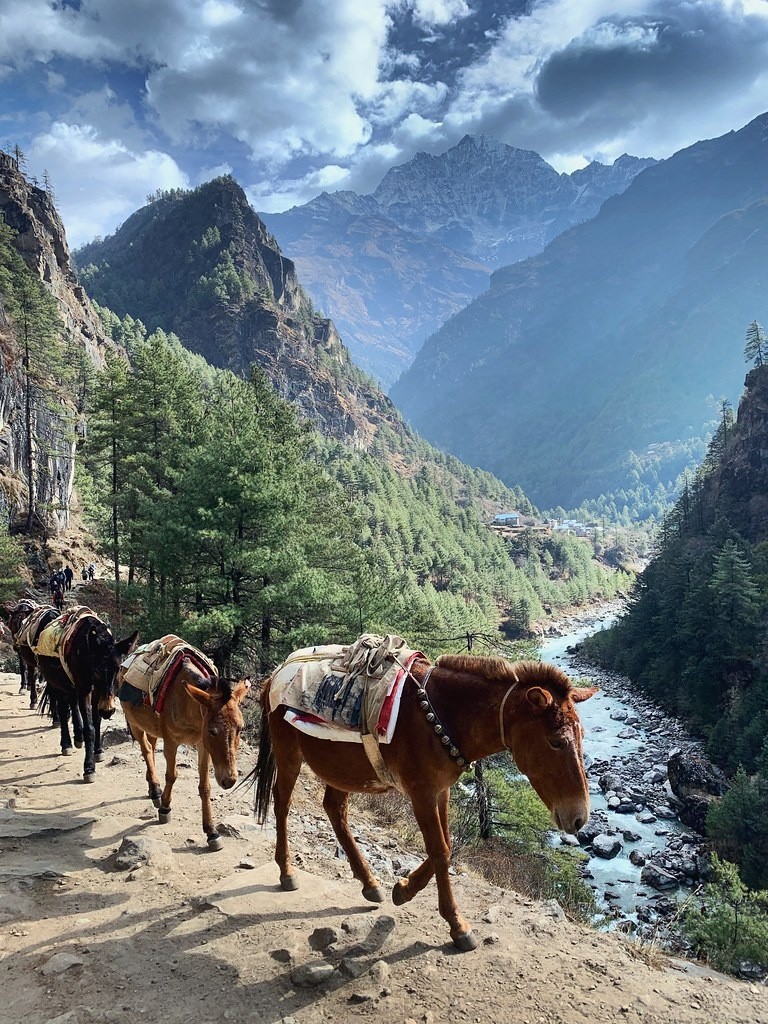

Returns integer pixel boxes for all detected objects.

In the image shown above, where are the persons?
[53,586,64,611]
[80,567,90,580]
[48,568,66,595]
[63,565,74,591]
[88,566,96,580]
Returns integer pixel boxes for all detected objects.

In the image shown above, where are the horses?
[253,633,599,951]
[0,590,138,783]
[115,633,250,851]
